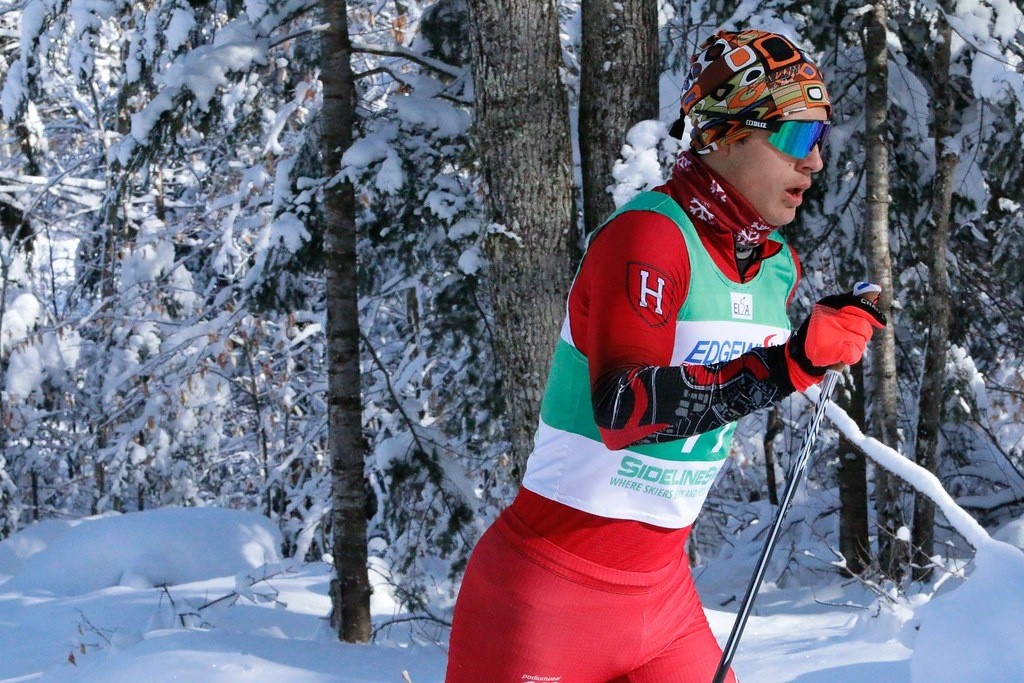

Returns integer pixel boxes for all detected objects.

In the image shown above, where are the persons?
[446,30,886,682]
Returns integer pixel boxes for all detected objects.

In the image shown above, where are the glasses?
[767,119,831,160]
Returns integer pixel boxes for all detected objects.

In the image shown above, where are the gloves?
[784,285,887,393]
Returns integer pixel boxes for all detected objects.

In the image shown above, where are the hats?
[668,30,832,154]
